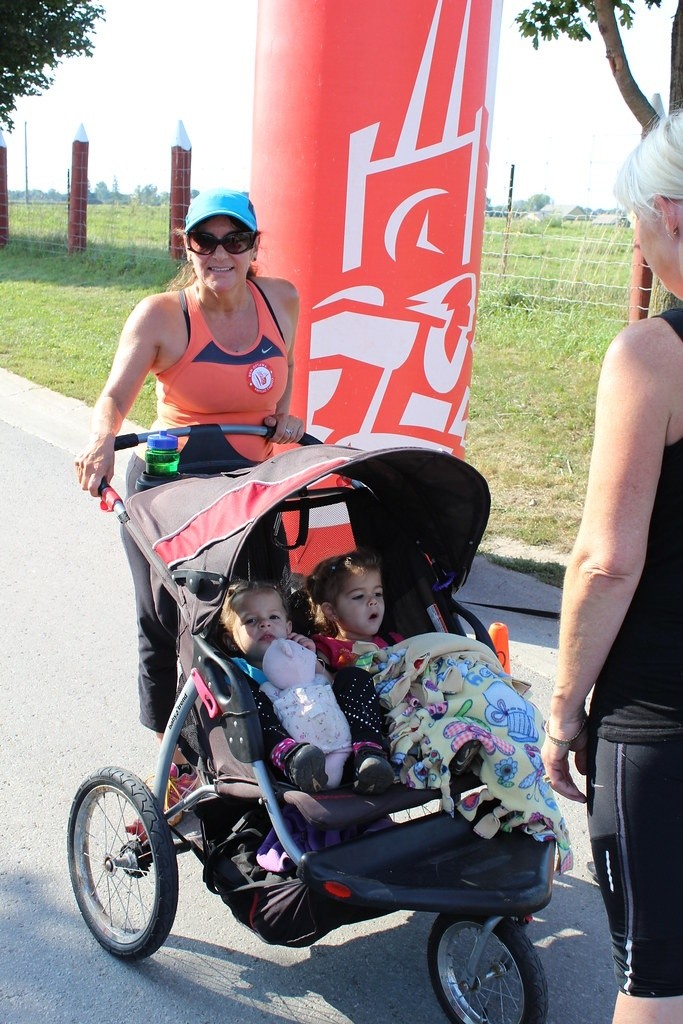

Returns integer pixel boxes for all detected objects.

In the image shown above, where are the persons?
[540,110,683,1024]
[217,550,483,796]
[75,188,304,826]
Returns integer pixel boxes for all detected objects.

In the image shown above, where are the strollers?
[66,424,555,1024]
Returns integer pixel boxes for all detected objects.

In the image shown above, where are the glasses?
[187,231,257,256]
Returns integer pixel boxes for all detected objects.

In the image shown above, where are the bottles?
[145,430,181,478]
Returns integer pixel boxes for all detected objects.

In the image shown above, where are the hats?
[185,188,257,235]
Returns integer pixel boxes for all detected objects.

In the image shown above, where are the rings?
[286,429,294,434]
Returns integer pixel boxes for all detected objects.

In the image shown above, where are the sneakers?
[167,762,200,812]
[284,742,329,793]
[450,739,482,776]
[351,746,395,796]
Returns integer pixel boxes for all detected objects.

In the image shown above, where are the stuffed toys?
[258,637,351,790]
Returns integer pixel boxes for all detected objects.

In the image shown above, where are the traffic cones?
[488,623,511,677]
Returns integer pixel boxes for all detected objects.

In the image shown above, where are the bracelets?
[318,659,325,674]
[544,713,588,747]
